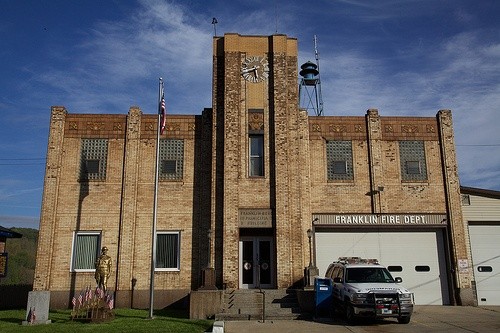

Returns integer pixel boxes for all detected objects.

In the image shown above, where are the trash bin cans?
[313,277,333,308]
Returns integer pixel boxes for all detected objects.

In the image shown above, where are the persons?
[95,246,113,295]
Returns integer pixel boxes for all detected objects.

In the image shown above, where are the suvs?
[324,257,415,322]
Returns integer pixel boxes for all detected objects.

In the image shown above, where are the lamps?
[378,185,384,214]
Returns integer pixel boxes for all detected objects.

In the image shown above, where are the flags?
[159,82,167,134]
[72,284,114,310]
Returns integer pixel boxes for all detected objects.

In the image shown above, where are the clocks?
[240,56,270,84]
